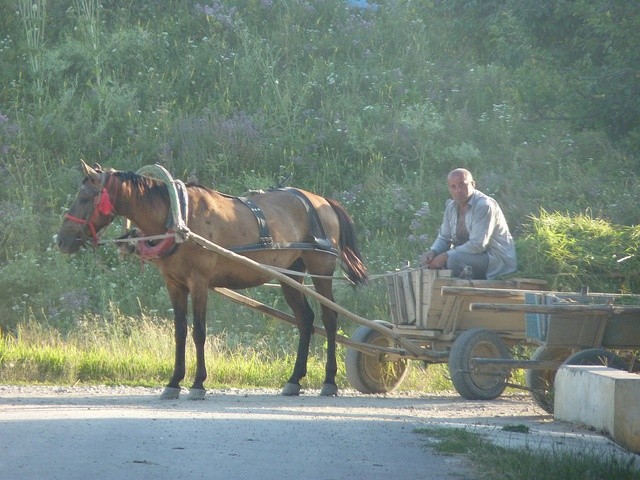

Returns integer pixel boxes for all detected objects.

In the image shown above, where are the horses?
[56,158,369,401]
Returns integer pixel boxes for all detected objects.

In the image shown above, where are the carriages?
[57,159,639,399]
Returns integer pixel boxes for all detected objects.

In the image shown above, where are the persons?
[421,168,519,280]
